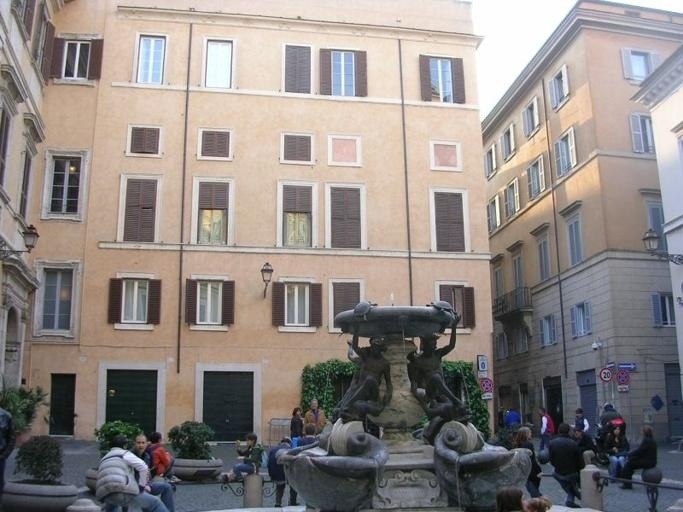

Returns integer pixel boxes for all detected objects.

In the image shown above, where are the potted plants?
[0,435,78,512]
[168,421,223,481]
[85,420,144,495]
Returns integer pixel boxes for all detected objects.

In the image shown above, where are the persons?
[267,399,327,508]
[223,433,264,482]
[0,407,16,499]
[496,402,657,512]
[406,312,466,422]
[96,430,178,512]
[330,326,393,422]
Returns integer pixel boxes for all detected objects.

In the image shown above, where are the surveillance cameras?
[592,343,598,351]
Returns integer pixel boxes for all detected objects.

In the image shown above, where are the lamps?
[642,229,683,266]
[0,225,40,260]
[261,263,273,297]
